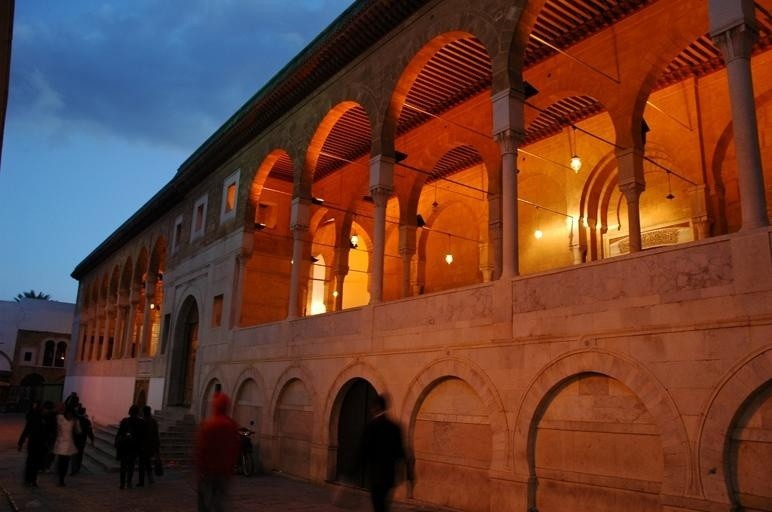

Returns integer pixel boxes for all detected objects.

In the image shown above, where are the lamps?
[445,235,453,264]
[351,214,358,247]
[431,182,439,213]
[667,171,675,200]
[570,126,582,174]
[534,206,543,240]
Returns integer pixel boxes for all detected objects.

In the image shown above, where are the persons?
[191,392,241,512]
[363,395,418,512]
[17,393,95,487]
[137,406,161,487]
[113,405,142,490]
